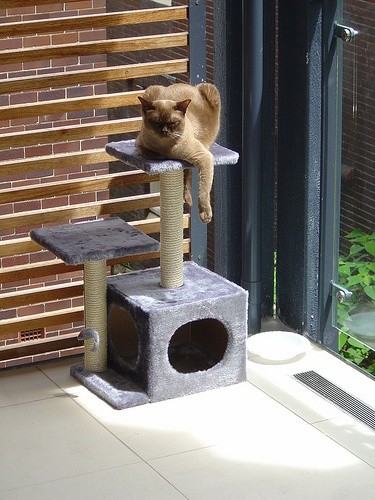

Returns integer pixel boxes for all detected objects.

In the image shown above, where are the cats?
[135,82,221,224]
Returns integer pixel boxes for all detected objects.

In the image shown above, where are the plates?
[246,331,311,360]
